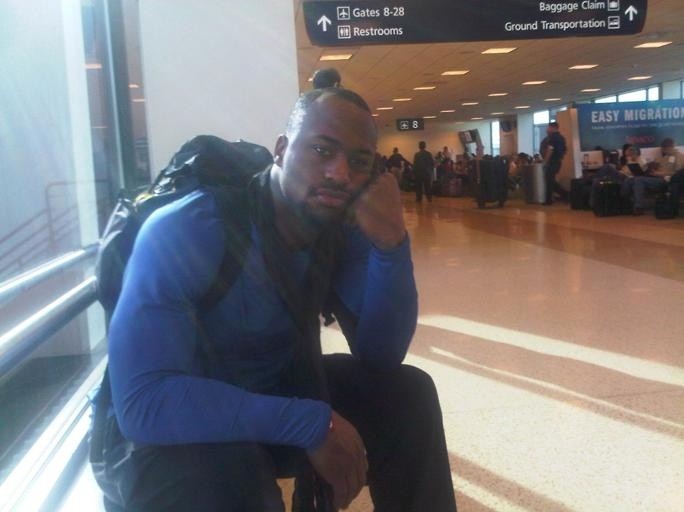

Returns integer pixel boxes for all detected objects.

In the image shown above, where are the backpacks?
[91,133,347,462]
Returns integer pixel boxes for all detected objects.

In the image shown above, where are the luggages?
[570,153,593,210]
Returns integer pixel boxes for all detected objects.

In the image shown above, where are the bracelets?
[328,404,337,430]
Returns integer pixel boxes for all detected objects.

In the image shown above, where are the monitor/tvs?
[500,121,512,133]
[462,132,476,143]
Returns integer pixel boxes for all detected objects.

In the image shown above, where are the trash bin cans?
[522,163,546,205]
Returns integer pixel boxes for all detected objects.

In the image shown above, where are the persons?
[345,28,348,36]
[616,138,682,215]
[87,88,461,512]
[375,141,541,203]
[540,121,567,204]
[339,28,343,36]
[312,67,340,89]
